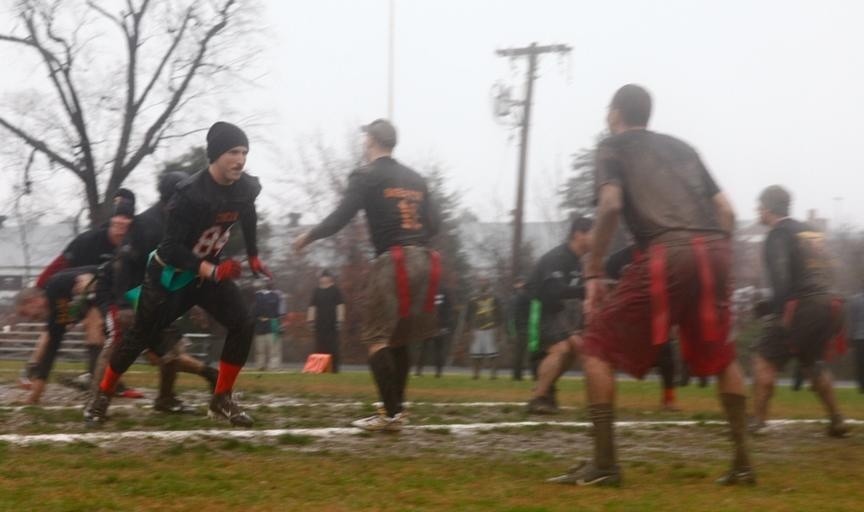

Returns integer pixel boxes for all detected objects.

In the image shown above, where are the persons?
[654,337,684,414]
[305,268,347,373]
[513,217,597,413]
[463,282,505,380]
[288,118,441,431]
[745,184,851,436]
[503,276,543,381]
[412,282,454,377]
[542,82,755,488]
[844,279,864,396]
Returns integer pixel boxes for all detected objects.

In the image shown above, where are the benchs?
[0,322,214,364]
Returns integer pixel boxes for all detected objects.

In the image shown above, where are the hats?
[111,188,134,202]
[205,121,249,166]
[157,172,188,198]
[359,119,398,149]
[109,205,133,220]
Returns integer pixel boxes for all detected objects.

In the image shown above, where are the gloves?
[248,256,272,280]
[211,260,245,283]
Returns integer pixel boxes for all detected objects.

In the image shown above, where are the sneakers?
[659,403,859,438]
[150,397,183,413]
[14,368,146,400]
[351,408,406,432]
[546,464,621,488]
[82,389,111,423]
[712,470,757,488]
[205,395,254,427]
[524,386,564,415]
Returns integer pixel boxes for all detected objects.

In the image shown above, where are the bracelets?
[582,274,604,281]
